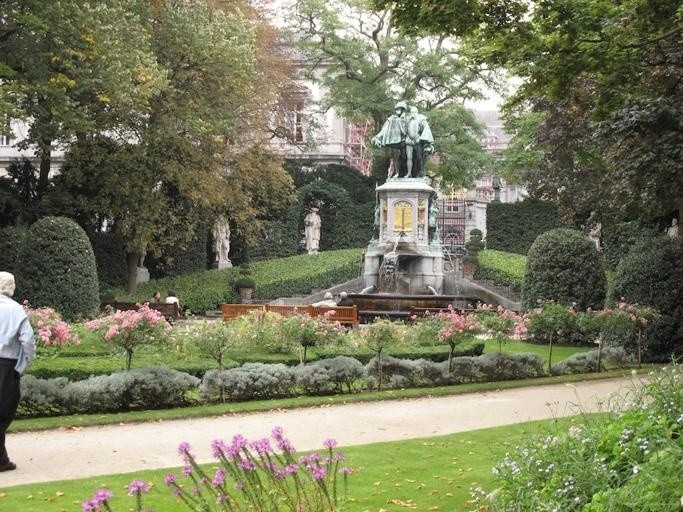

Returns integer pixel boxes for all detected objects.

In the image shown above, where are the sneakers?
[0,462,16,471]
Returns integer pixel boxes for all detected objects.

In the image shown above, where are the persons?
[213,212,232,263]
[406,106,433,178]
[590,211,602,251]
[0,271,36,470]
[371,101,407,177]
[338,292,360,325]
[304,206,322,255]
[150,292,160,303]
[309,292,336,307]
[668,217,679,238]
[166,289,180,312]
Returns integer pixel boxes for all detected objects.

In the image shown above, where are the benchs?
[100,301,188,325]
[457,310,519,317]
[311,304,362,328]
[406,306,457,324]
[220,301,266,323]
[358,311,410,325]
[266,302,313,320]
